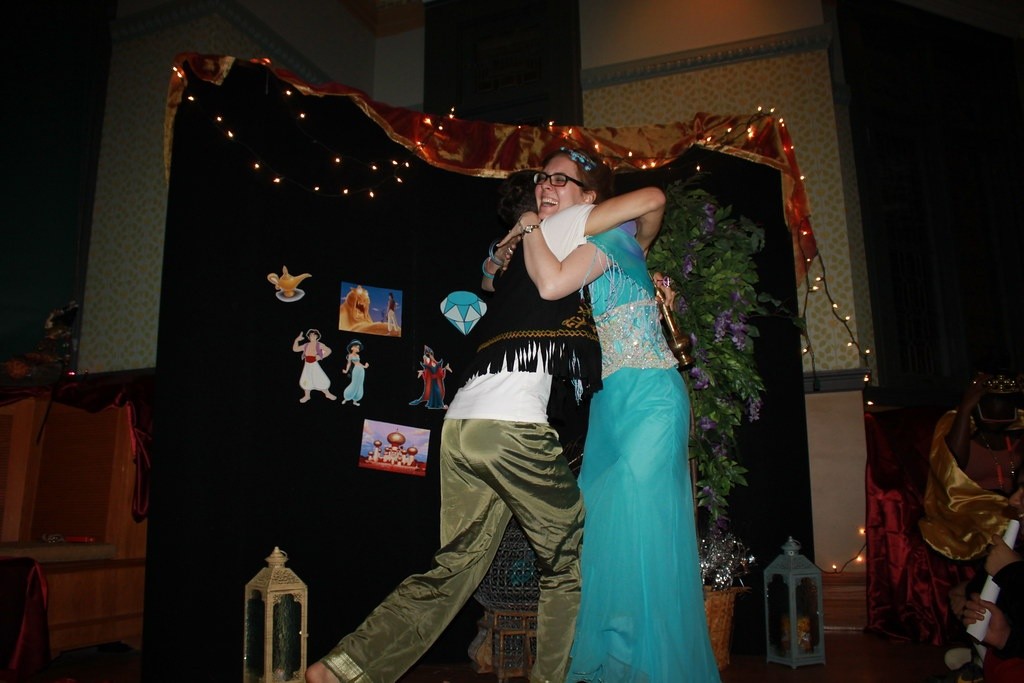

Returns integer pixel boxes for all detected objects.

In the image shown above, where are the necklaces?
[959,462,1024,682]
[972,423,1016,494]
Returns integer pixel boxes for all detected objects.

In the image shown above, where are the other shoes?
[943,642,987,672]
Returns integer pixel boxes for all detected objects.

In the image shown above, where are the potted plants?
[641,175,799,680]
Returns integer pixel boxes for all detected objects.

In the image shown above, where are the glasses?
[534,172,584,188]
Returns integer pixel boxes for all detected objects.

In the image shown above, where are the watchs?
[521,223,541,239]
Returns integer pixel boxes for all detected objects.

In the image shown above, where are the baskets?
[701,585,752,673]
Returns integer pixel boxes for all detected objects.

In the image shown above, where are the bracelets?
[488,238,505,266]
[480,256,496,279]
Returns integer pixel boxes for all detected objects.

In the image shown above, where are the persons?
[918,371,1024,567]
[303,165,670,682]
[493,147,722,683]
